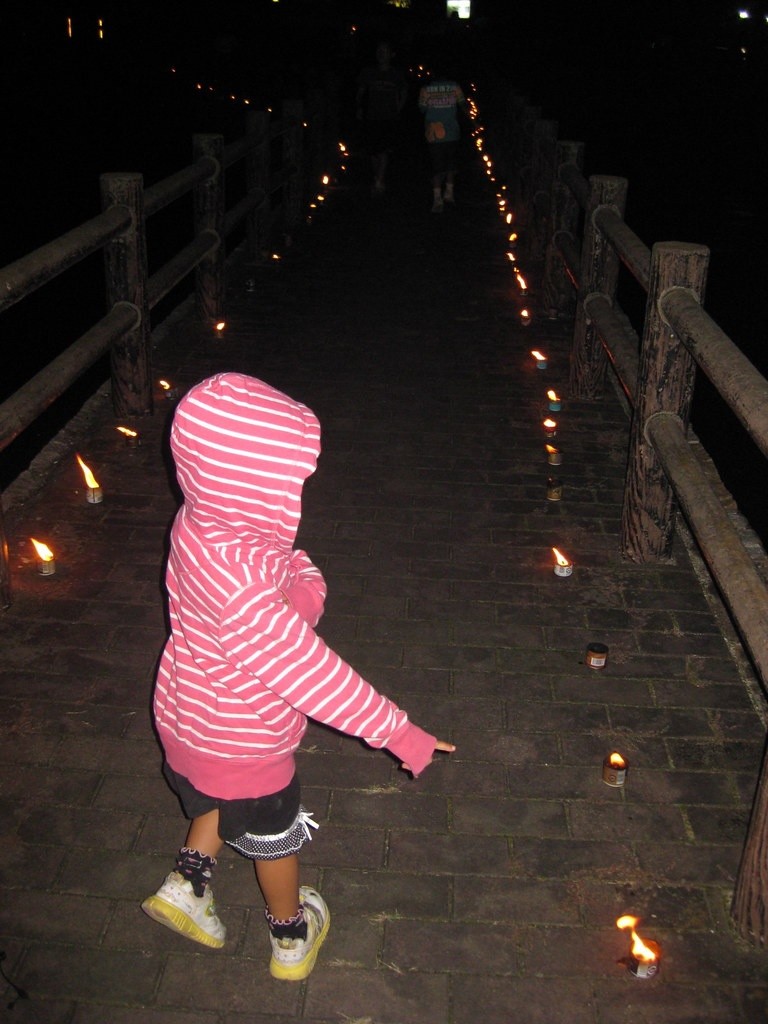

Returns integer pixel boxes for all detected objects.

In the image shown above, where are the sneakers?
[141,872,229,949]
[270,885,332,980]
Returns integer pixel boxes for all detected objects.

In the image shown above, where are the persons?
[418,63,467,215]
[355,37,413,196]
[141,371,456,982]
